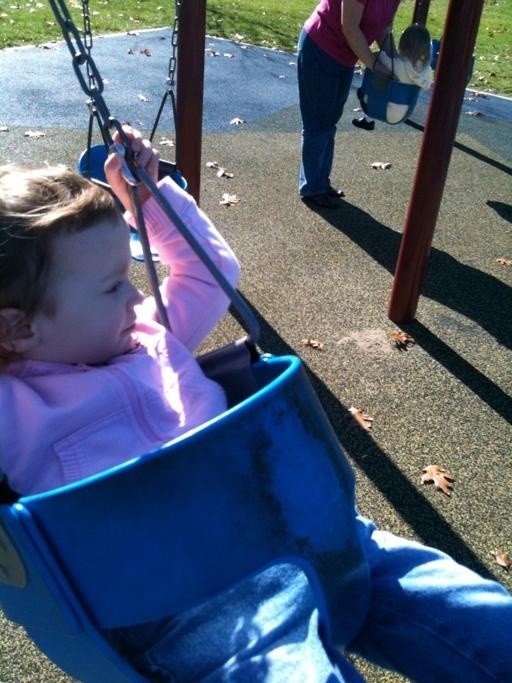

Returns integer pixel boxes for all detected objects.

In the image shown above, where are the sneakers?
[357,88,361,97]
[352,117,375,130]
[302,187,343,208]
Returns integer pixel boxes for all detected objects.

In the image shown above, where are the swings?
[0,2,372,683]
[359,1,476,125]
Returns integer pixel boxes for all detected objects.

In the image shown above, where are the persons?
[352,23,434,130]
[0,125,511,683]
[296,0,400,209]
